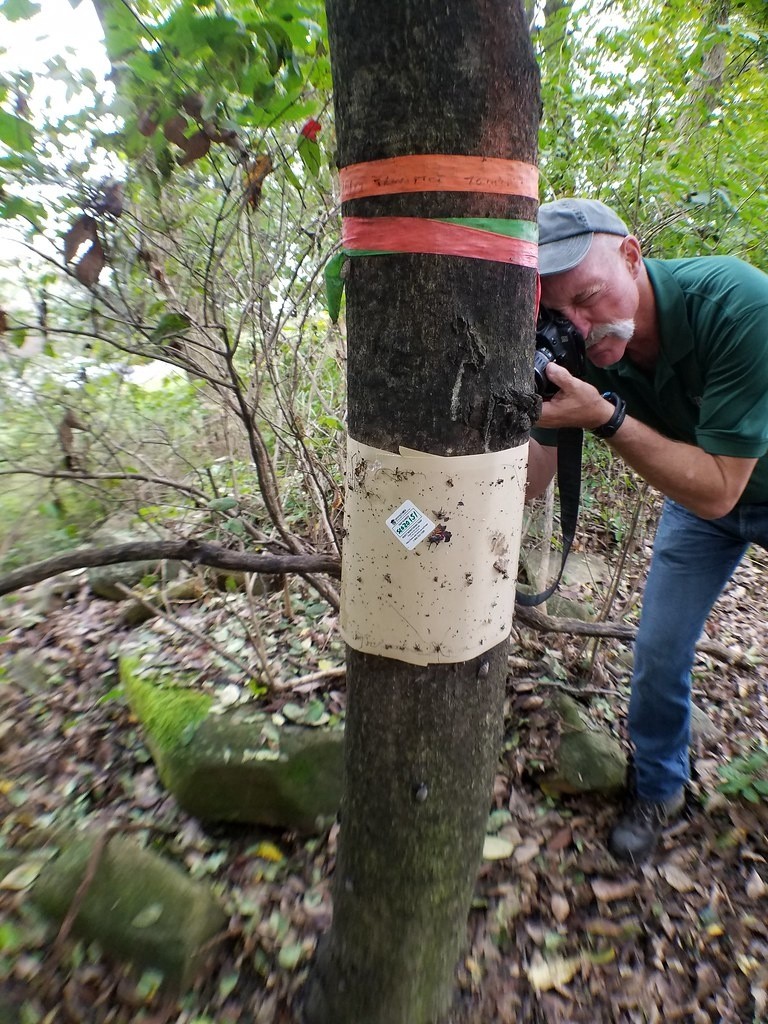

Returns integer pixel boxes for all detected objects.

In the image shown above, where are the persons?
[535,197,768,861]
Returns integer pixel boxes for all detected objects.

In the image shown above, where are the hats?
[537,197,630,277]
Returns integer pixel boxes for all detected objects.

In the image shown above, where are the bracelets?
[588,389,629,442]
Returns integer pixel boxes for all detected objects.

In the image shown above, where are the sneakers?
[611,786,686,858]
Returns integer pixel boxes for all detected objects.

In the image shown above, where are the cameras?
[532,310,587,399]
